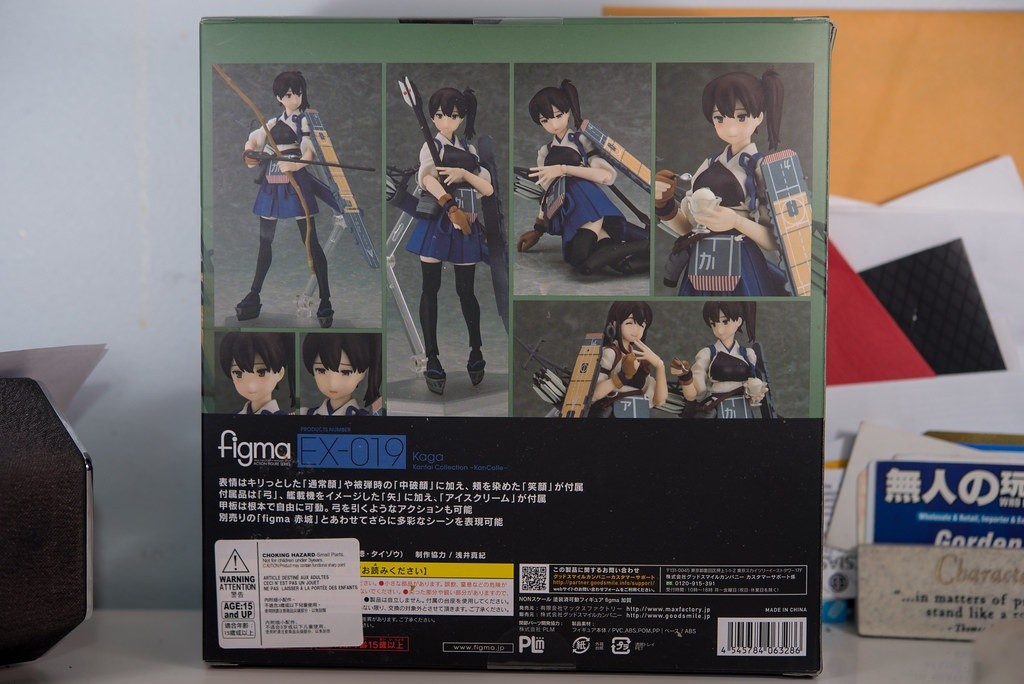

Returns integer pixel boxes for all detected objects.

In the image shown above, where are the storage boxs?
[853,469,1023,643]
